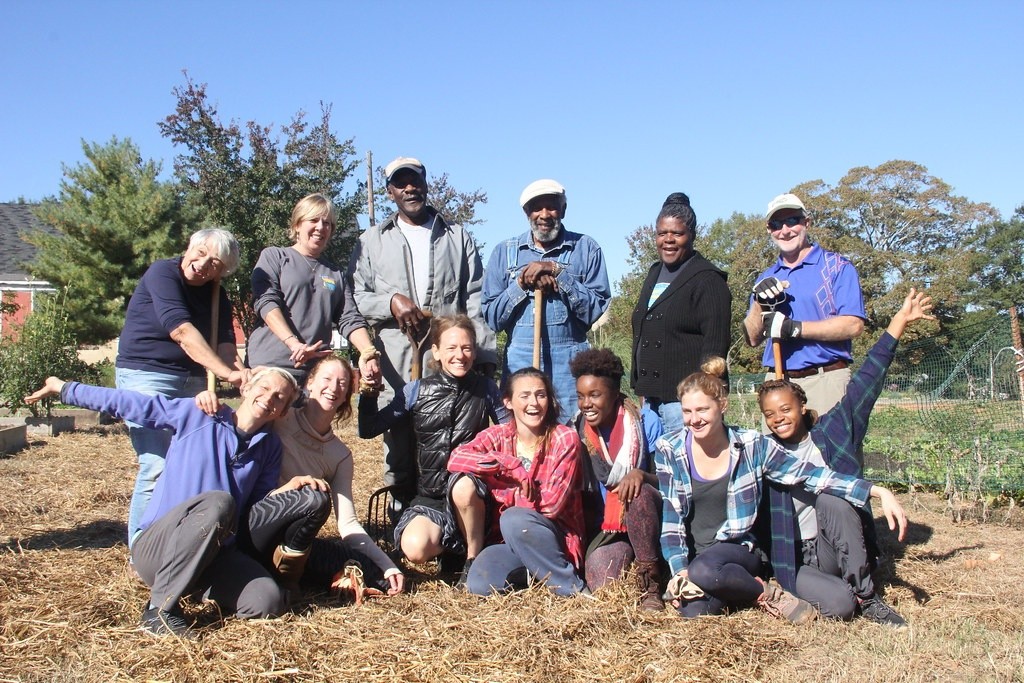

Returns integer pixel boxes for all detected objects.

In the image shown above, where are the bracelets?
[551,261,556,275]
[283,335,297,344]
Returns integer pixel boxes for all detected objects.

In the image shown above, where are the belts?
[772,360,850,379]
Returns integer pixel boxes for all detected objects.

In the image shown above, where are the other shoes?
[435,551,476,592]
[143,600,200,645]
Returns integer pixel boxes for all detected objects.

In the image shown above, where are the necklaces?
[292,246,318,272]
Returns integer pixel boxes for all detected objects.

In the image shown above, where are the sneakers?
[853,591,907,631]
[757,577,818,629]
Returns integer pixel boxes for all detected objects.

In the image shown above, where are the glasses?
[767,215,803,230]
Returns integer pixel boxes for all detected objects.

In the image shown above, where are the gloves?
[760,312,803,341]
[751,275,791,302]
[661,574,706,601]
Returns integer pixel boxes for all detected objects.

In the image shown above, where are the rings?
[406,321,412,326]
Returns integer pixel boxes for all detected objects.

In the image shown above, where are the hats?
[764,194,809,220]
[520,178,569,212]
[383,156,428,185]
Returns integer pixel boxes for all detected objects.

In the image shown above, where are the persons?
[564,347,665,612]
[243,193,382,408]
[344,156,497,526]
[480,178,612,425]
[629,193,733,435]
[195,355,406,596]
[653,356,908,627]
[741,194,868,436]
[749,288,935,627]
[357,314,513,591]
[446,366,594,604]
[23,367,298,641]
[115,228,266,568]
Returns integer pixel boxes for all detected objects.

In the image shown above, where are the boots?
[632,556,671,619]
[270,542,312,607]
